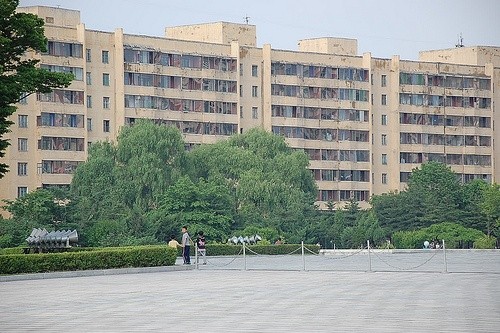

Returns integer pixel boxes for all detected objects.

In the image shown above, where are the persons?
[275,238,282,245]
[168,234,182,265]
[182,226,194,265]
[194,231,207,265]
